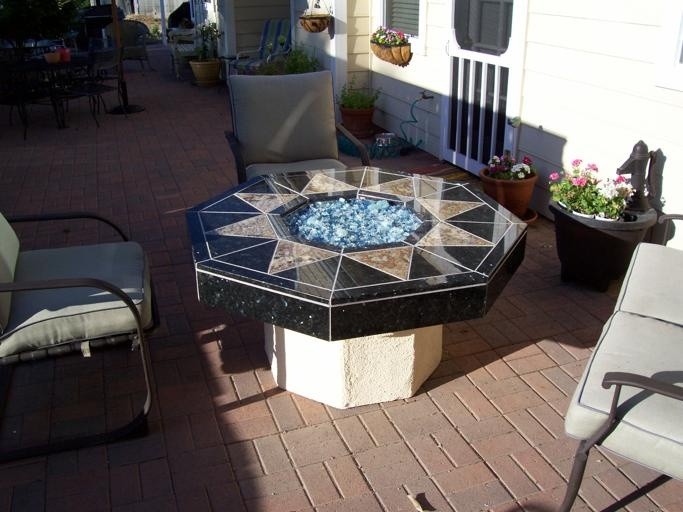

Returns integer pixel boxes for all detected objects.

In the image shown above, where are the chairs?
[50,58,103,132]
[558,236,683,512]
[15,60,63,142]
[225,71,370,185]
[230,17,291,75]
[67,46,129,129]
[0,209,160,465]
[0,62,26,143]
[121,20,153,72]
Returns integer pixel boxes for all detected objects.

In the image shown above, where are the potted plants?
[336,74,381,140]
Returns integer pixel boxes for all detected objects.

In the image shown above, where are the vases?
[546,198,657,298]
[479,167,538,220]
[188,58,221,88]
[370,41,410,66]
[299,13,331,34]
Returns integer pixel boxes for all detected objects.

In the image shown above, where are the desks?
[185,165,528,411]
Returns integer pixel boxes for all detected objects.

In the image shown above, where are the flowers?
[486,149,536,180]
[545,159,637,219]
[194,23,223,61]
[370,26,408,47]
